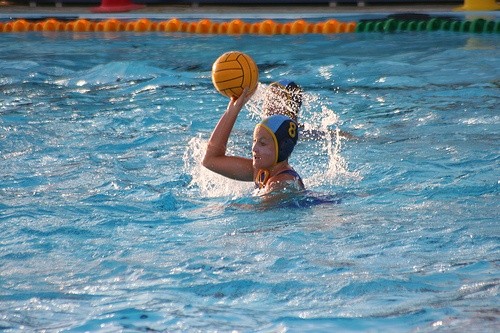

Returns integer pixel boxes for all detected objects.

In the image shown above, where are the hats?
[256,114,298,166]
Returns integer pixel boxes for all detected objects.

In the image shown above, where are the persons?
[260,79,359,141]
[201,83,359,211]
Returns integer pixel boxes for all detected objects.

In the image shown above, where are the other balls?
[211,52,258,100]
